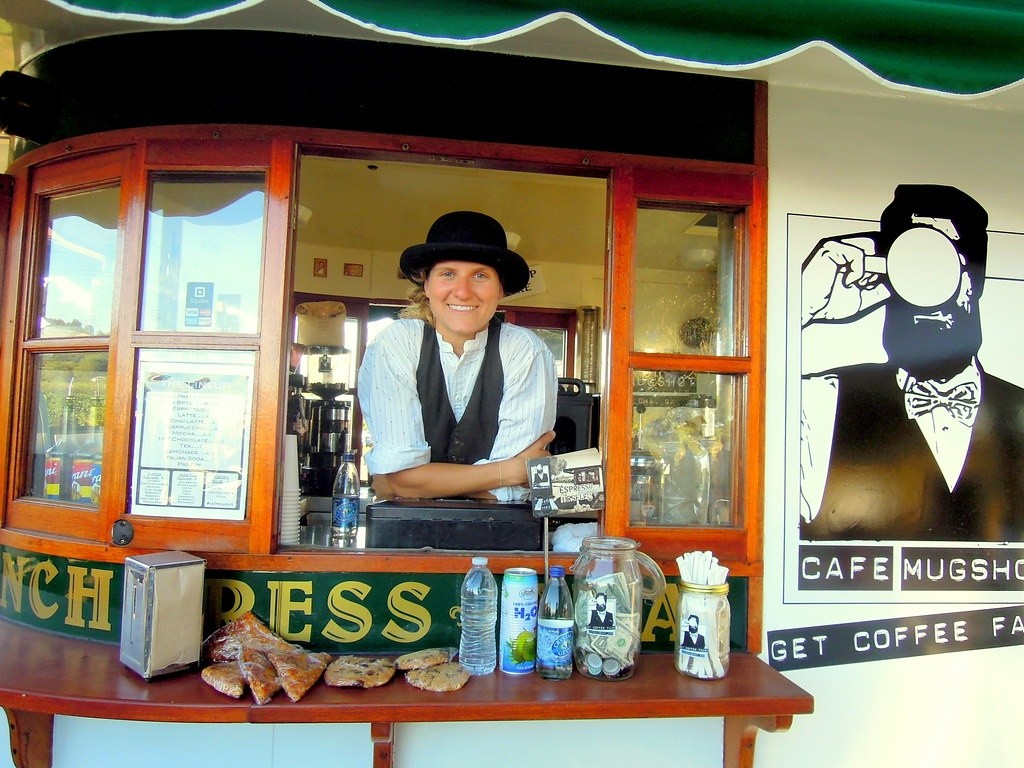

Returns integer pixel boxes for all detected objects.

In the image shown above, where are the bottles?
[459,556,498,676]
[571,536,666,681]
[537,566,575,681]
[45,397,103,504]
[674,580,731,680]
[330,455,360,539]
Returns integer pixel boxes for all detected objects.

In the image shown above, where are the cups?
[280,435,301,545]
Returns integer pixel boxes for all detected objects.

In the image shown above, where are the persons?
[356,211,560,501]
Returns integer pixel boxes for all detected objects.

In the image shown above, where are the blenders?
[305,347,353,468]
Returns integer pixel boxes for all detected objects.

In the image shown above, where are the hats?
[399,210,530,298]
